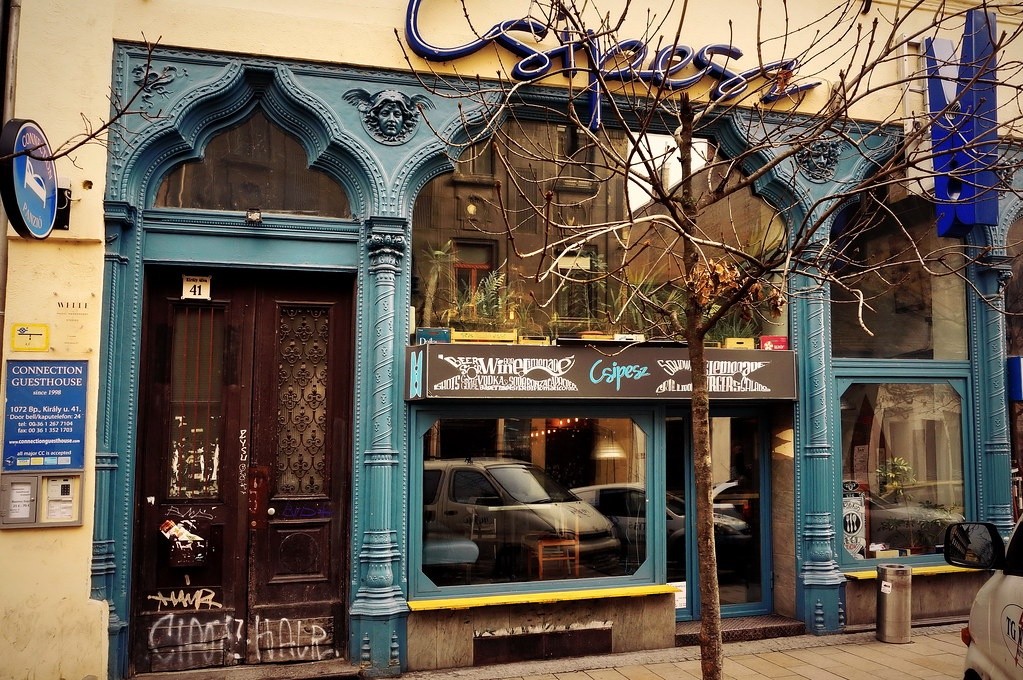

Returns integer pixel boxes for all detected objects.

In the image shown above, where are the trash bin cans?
[876,564,913,644]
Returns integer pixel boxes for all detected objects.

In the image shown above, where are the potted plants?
[874,457,925,555]
[442,261,764,349]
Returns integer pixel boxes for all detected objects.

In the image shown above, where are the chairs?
[467,504,581,581]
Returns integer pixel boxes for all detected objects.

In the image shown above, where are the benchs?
[845,566,985,580]
[406,584,682,612]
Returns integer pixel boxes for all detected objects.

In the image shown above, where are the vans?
[423,456,623,575]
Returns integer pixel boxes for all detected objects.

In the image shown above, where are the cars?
[843,479,965,552]
[943,511,1022,679]
[711,480,760,516]
[568,482,753,569]
